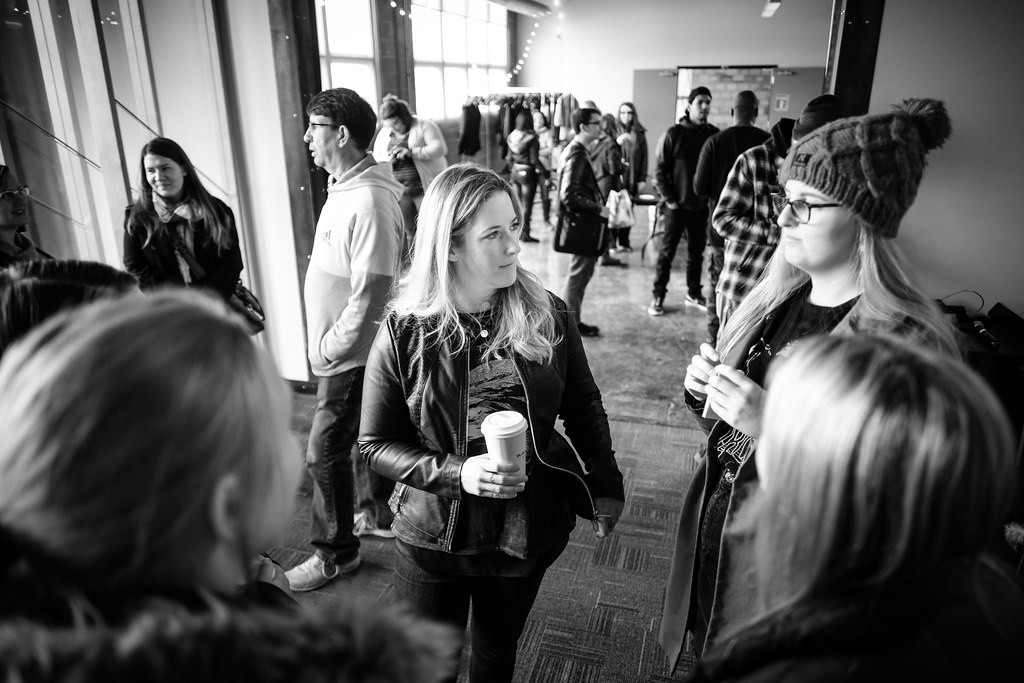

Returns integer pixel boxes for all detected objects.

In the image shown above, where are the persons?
[506,111,557,243]
[710,94,857,331]
[0,257,143,354]
[372,94,448,253]
[123,138,243,296]
[656,99,955,678]
[0,164,53,265]
[282,88,407,593]
[693,90,770,344]
[553,100,648,337]
[698,331,1024,683]
[0,285,459,683]
[356,163,624,683]
[648,86,723,316]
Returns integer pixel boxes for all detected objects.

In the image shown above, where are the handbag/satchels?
[605,188,636,228]
[555,211,610,257]
[226,282,267,336]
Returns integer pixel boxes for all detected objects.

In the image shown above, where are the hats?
[685,86,713,114]
[778,97,951,239]
[791,94,850,141]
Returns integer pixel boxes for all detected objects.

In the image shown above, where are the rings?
[490,474,494,483]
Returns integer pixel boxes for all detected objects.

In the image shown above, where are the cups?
[480,409,529,488]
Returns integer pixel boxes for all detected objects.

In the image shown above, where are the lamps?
[760,0,781,19]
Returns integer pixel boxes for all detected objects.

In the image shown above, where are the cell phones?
[593,515,609,538]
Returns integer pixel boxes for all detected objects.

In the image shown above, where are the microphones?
[972,321,999,346]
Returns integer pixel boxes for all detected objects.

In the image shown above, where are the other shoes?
[619,243,632,253]
[544,220,555,231]
[685,292,710,312]
[607,245,618,254]
[648,294,664,316]
[601,256,622,266]
[576,322,600,337]
[519,232,538,242]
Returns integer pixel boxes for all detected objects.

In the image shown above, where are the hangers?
[467,92,563,109]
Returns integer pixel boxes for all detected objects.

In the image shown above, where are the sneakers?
[353,511,398,539]
[285,545,361,590]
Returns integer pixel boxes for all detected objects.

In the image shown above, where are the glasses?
[768,190,842,224]
[308,122,342,135]
[581,119,603,127]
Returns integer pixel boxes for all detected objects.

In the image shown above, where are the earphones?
[479,324,488,337]
